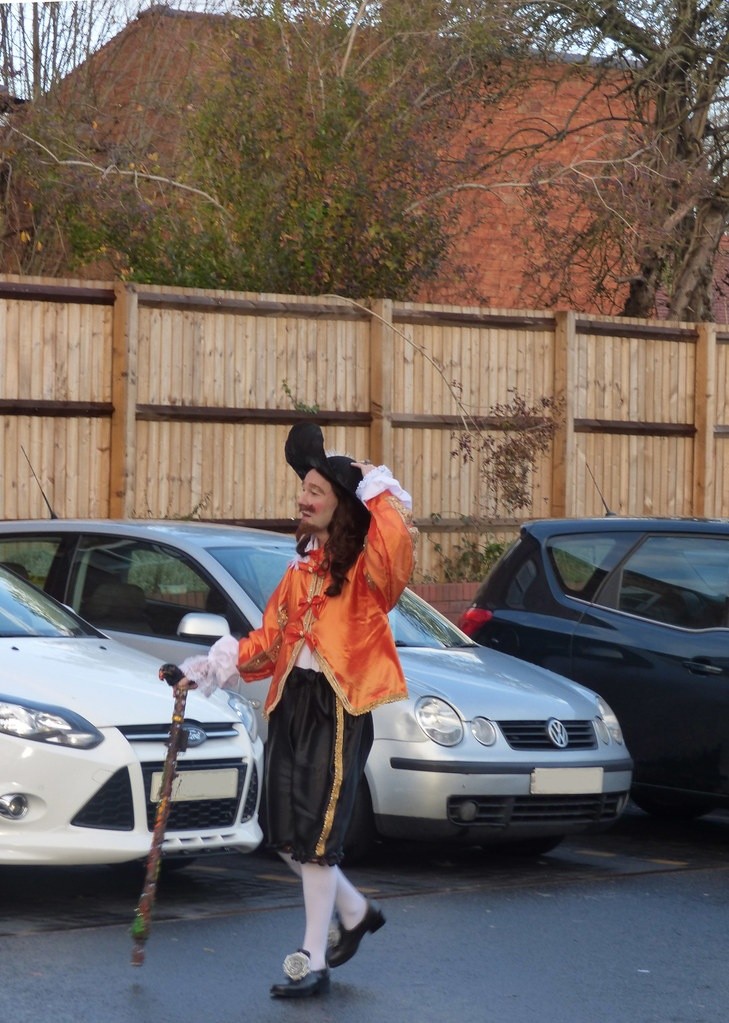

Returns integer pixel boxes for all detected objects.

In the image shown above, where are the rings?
[364,459,370,464]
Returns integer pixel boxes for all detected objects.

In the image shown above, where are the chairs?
[81,583,155,637]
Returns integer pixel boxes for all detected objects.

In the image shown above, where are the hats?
[285,422,363,497]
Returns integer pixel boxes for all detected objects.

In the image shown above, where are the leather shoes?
[326,899,387,967]
[270,949,330,998]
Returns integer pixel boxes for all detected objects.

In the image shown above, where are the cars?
[459,517,729,819]
[1,563,263,864]
[0,520,634,869]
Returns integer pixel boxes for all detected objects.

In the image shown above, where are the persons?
[177,421,415,998]
